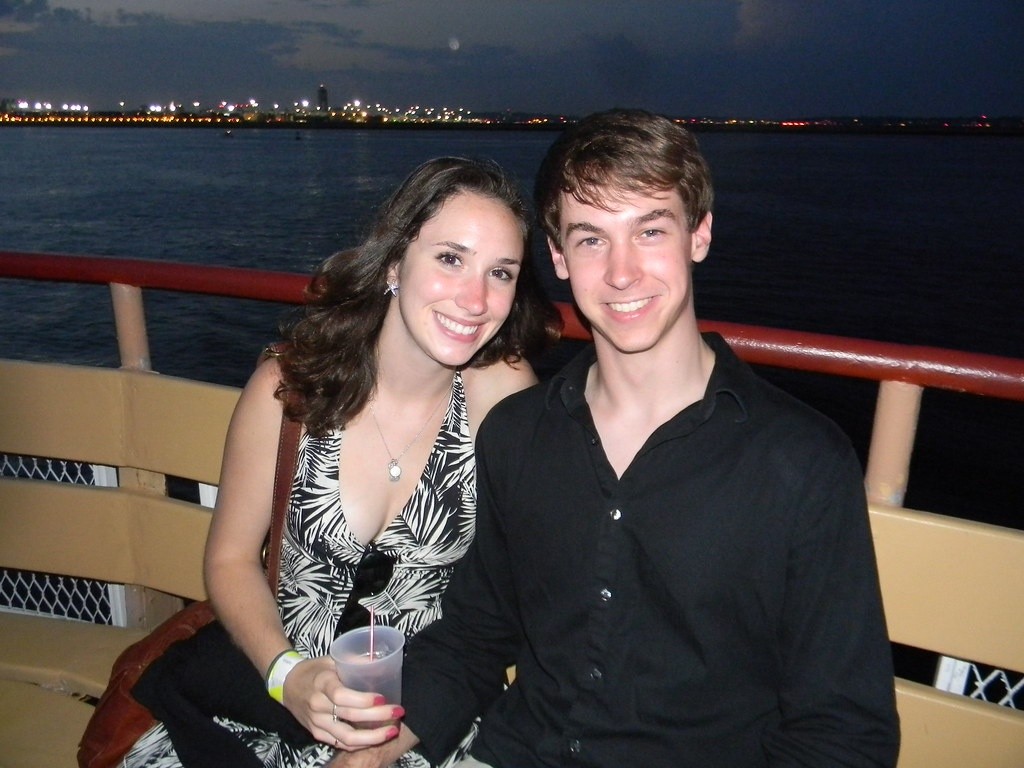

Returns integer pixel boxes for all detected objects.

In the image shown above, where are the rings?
[333,706,337,723]
[335,739,338,746]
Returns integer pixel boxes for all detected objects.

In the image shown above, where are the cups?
[330,626,405,730]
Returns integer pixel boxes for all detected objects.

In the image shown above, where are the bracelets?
[263,649,306,705]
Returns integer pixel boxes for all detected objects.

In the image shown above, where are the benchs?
[0,358,1024,768]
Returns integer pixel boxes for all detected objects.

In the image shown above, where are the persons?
[117,152,539,768]
[365,383,452,482]
[322,108,900,768]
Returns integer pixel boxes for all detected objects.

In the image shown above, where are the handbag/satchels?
[77,600,216,768]
